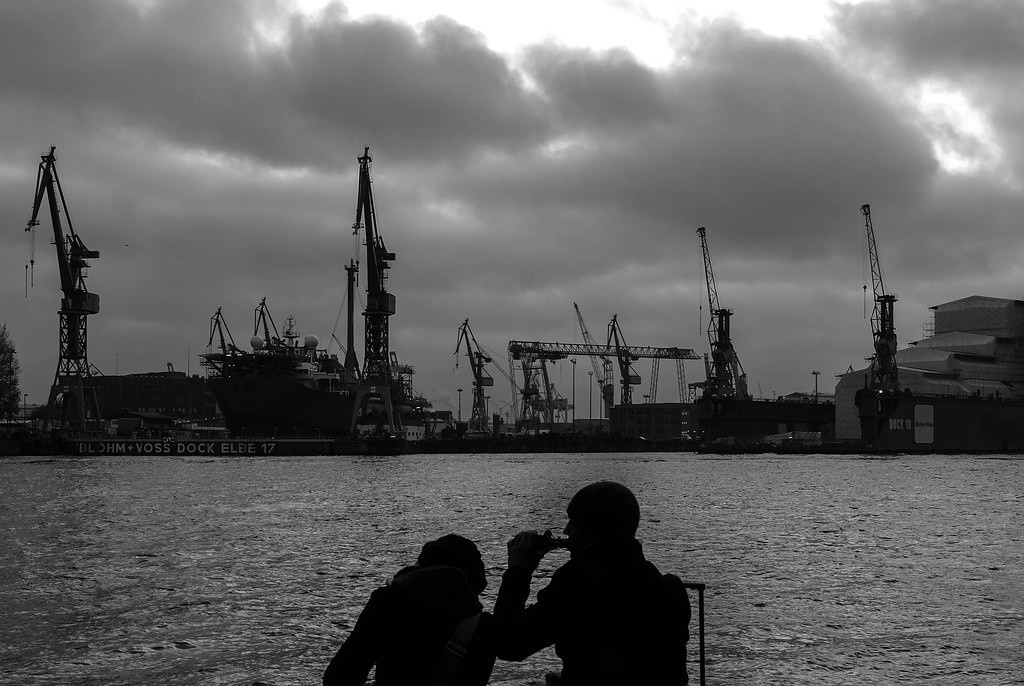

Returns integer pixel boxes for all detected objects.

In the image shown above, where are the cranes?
[454,317,494,435]
[26,146,103,436]
[351,146,407,443]
[571,302,613,409]
[606,315,641,404]
[697,227,751,439]
[859,202,902,396]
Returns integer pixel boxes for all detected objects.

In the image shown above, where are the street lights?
[570,357,576,435]
[587,371,594,434]
[23,394,29,424]
[456,388,463,435]
[598,380,605,435]
[811,369,821,407]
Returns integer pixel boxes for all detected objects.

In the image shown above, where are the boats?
[198,314,362,439]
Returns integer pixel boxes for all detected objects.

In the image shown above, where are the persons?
[492,481,691,686]
[323,534,496,686]
